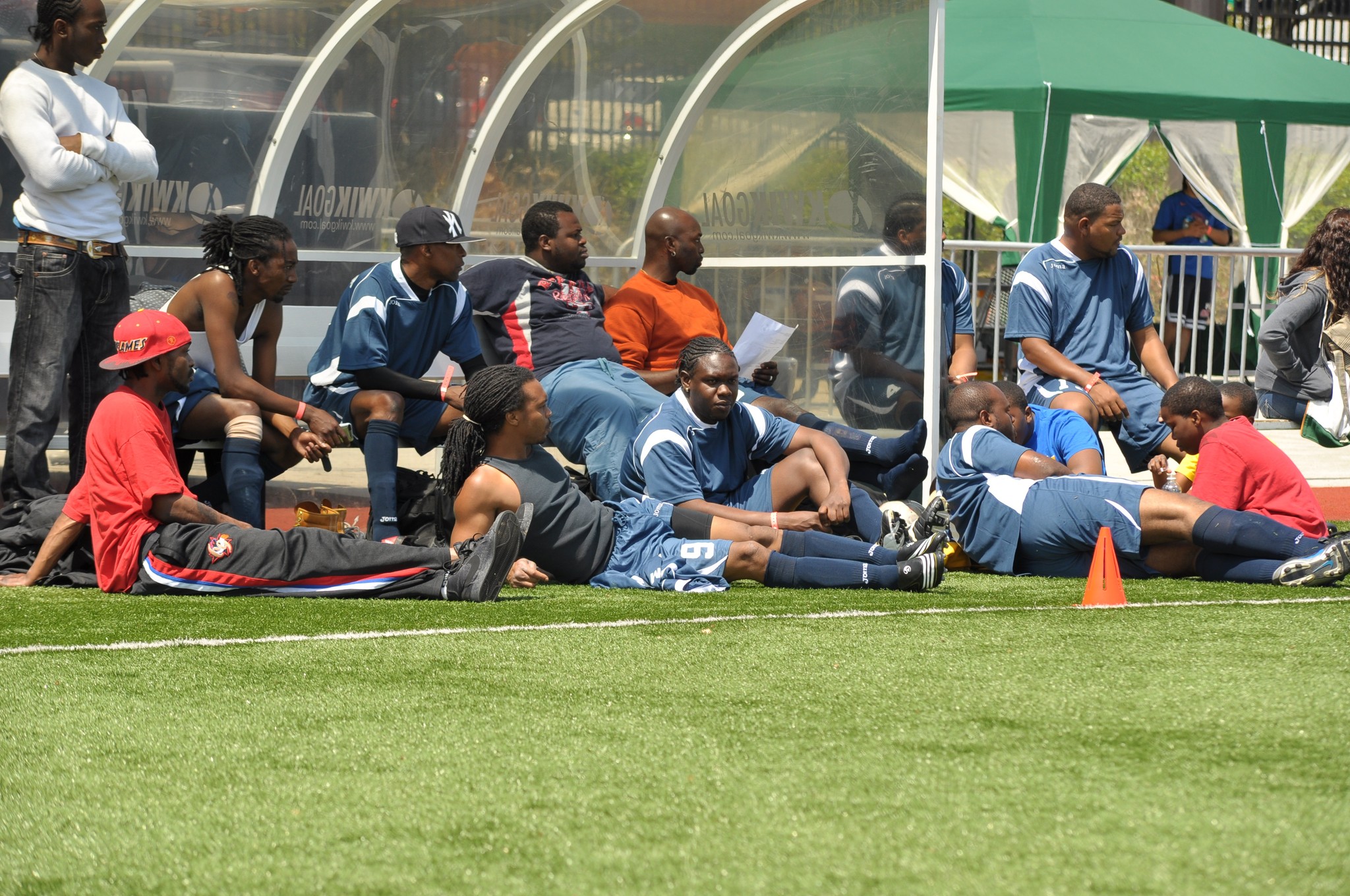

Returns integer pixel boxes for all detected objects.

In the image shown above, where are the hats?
[99,309,192,370]
[394,205,487,248]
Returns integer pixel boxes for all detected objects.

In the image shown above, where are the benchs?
[178,432,455,458]
[1097,417,1297,432]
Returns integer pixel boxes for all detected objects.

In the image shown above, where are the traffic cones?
[1072,527,1129,607]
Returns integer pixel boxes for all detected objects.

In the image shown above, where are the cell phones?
[336,422,354,444]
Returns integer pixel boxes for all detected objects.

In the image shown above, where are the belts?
[18,231,129,260]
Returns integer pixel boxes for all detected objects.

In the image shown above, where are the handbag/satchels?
[366,466,454,548]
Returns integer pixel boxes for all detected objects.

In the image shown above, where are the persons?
[618,337,947,552]
[602,207,928,501]
[1255,207,1349,422]
[1152,174,1233,321]
[1,0,159,495]
[159,215,350,529]
[459,201,671,500]
[1,0,1350,602]
[1004,183,1188,473]
[437,364,946,593]
[301,206,487,544]
[1,309,522,602]
[827,194,977,430]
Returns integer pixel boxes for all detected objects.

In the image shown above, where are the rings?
[770,376,772,381]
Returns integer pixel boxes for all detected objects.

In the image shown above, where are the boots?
[293,498,348,534]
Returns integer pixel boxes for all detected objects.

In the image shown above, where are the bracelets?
[1207,225,1212,236]
[295,401,306,420]
[1084,372,1101,392]
[955,372,977,382]
[769,512,778,530]
[440,365,454,401]
[288,427,311,441]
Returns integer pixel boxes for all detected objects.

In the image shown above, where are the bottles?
[1161,470,1182,493]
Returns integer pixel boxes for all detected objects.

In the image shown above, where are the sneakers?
[442,502,534,574]
[447,511,521,604]
[897,532,950,561]
[897,550,948,591]
[876,509,909,550]
[906,496,951,542]
[1272,531,1350,586]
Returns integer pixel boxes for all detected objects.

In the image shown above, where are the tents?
[661,1,1349,346]
